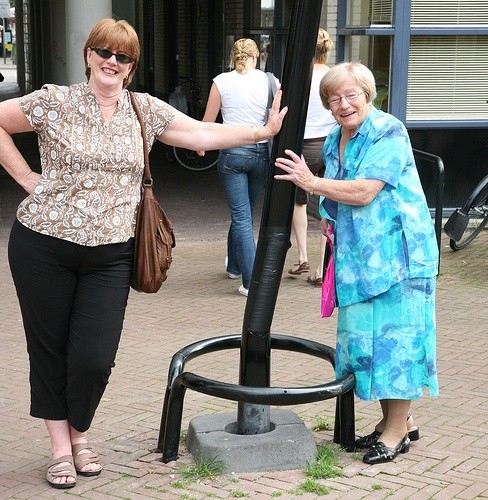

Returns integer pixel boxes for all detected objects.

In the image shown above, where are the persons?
[274,62,439,464]
[0,17,289,489]
[195,38,281,298]
[289,26,341,287]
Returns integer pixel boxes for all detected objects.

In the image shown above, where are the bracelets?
[309,176,320,196]
[251,125,261,143]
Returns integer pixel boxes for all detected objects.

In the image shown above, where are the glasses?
[91,47,133,64]
[328,90,365,107]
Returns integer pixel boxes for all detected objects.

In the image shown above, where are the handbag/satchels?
[131,187,176,293]
[320,221,335,318]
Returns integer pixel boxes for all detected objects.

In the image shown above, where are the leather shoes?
[363,431,410,464]
[356,413,419,448]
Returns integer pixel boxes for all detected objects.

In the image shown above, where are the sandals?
[72,443,102,477]
[289,260,310,275]
[46,455,77,489]
[308,275,322,286]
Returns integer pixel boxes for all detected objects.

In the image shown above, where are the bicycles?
[169,76,222,171]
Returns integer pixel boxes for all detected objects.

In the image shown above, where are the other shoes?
[239,285,248,297]
[225,255,242,279]
